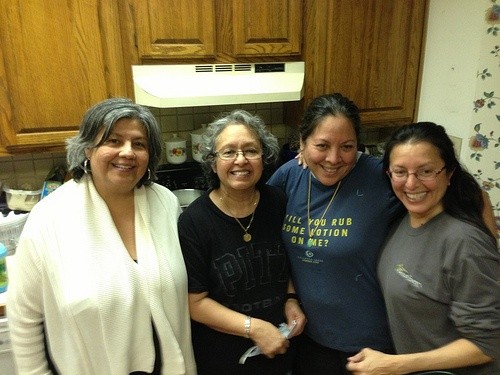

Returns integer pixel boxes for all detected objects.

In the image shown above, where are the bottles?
[0,241,9,293]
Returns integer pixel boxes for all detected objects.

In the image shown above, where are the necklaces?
[307,170,341,246]
[217,189,258,241]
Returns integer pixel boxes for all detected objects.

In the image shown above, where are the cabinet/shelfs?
[0,0,430,157]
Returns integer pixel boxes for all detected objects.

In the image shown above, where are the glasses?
[386,164,447,182]
[210,147,264,160]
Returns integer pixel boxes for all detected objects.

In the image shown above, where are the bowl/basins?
[3,180,42,211]
[171,189,205,212]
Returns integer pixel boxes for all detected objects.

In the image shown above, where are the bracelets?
[286,293,298,301]
[246,315,251,338]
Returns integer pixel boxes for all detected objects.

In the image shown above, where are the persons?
[273,94,496,375]
[295,122,500,375]
[6,99,197,375]
[178,110,306,375]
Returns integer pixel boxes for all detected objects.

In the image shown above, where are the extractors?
[131,60,306,108]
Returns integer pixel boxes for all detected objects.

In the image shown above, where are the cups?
[189,123,208,163]
[165,133,187,165]
